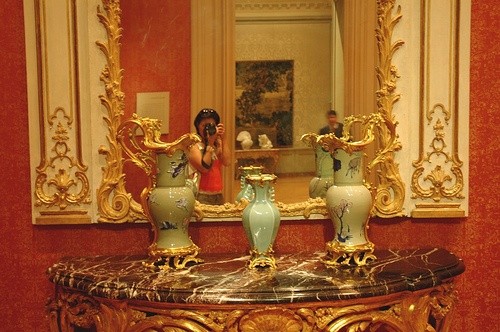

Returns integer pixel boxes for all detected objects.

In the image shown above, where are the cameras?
[204,123,218,137]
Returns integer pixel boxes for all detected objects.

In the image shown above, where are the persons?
[185,109,232,206]
[314,110,353,171]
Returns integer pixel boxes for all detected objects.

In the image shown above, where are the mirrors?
[234,1,347,204]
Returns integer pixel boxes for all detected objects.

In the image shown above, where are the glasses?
[200,109,216,114]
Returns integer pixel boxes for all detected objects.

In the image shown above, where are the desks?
[45,245,467,332]
